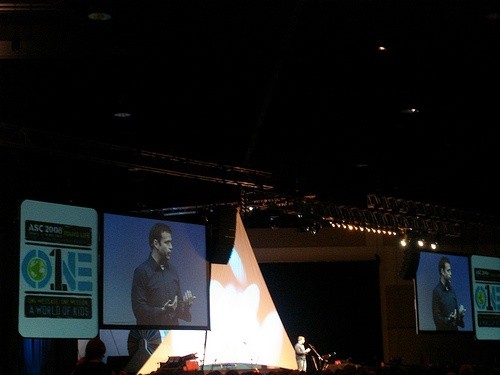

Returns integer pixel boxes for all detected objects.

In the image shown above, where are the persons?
[432,257,467,330]
[294,335,311,372]
[131,223,197,325]
[71,338,127,375]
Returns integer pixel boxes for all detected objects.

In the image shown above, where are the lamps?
[324,194,500,251]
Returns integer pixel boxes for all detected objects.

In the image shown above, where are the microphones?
[160,265,165,270]
[446,287,449,291]
